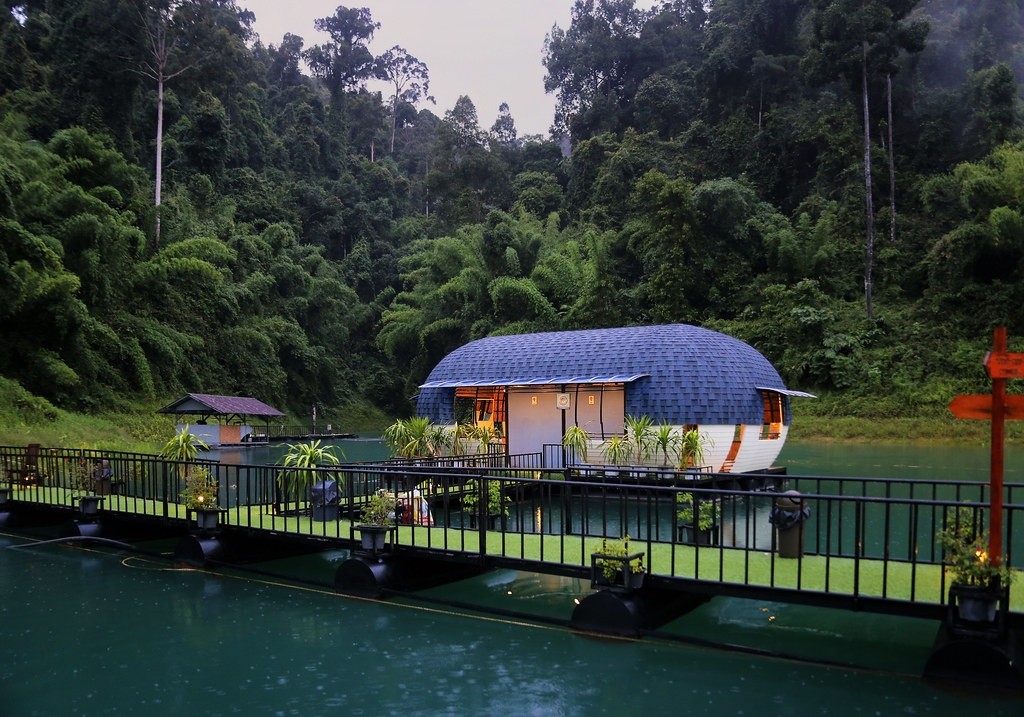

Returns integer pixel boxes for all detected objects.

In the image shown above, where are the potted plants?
[0,459,11,503]
[620,413,659,477]
[640,421,683,478]
[677,492,720,543]
[111,461,148,494]
[179,467,220,527]
[937,501,1014,620]
[594,534,646,590]
[681,428,705,479]
[358,486,398,550]
[63,460,103,512]
[595,433,634,476]
[560,420,597,475]
[459,481,512,528]
